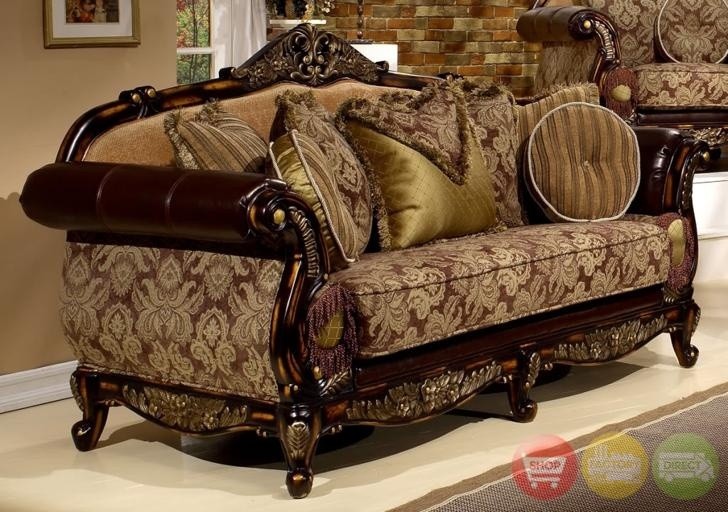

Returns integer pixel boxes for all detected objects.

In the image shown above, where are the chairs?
[514,0,728,174]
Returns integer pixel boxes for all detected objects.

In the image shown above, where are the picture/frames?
[41,0,143,50]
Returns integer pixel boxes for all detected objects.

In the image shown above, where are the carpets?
[382,381,728,511]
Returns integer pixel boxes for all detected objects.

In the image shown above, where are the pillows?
[656,1,728,66]
[163,65,648,275]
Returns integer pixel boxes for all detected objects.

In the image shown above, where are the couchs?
[17,71,714,499]
